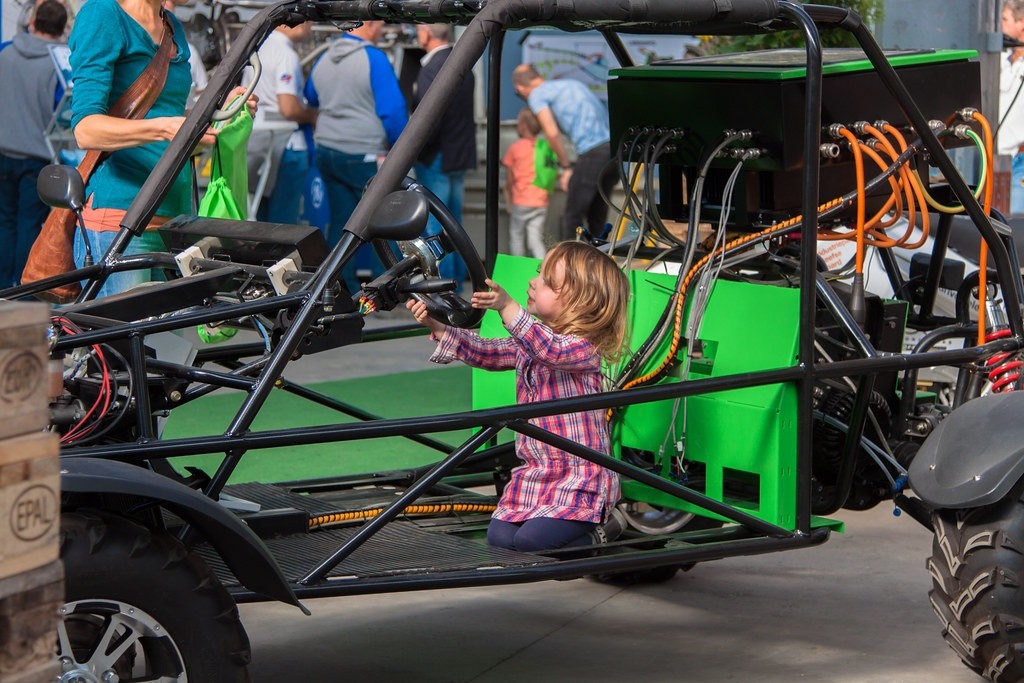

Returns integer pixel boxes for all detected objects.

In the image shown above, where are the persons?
[409,20,479,297]
[405,242,629,553]
[240,21,322,226]
[996,0,1024,216]
[65,0,260,299]
[511,62,620,243]
[302,20,414,295]
[0,0,67,304]
[502,109,554,260]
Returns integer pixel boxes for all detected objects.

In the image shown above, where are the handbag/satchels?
[21,208,80,302]
[198,94,254,343]
[530,137,560,195]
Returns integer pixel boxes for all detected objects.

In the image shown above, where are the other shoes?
[595,507,627,544]
